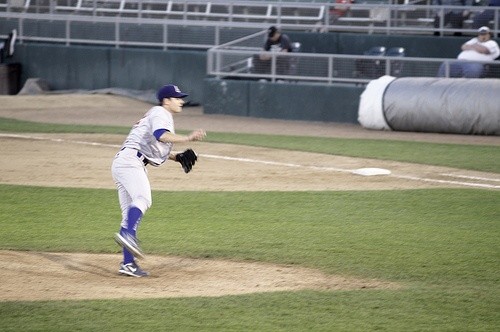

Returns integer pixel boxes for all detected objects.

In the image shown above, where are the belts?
[121,146,150,166]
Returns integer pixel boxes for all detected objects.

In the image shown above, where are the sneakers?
[114,231,144,260]
[118,263,149,278]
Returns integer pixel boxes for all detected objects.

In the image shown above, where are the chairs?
[356,47,405,87]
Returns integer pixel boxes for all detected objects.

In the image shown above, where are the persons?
[111,84,206,278]
[263,25,293,74]
[432,0,500,78]
[326,0,354,25]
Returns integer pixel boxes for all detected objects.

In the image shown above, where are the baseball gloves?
[175,149,198,174]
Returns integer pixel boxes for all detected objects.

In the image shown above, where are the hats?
[476,27,489,34]
[158,84,189,103]
[267,27,278,37]
